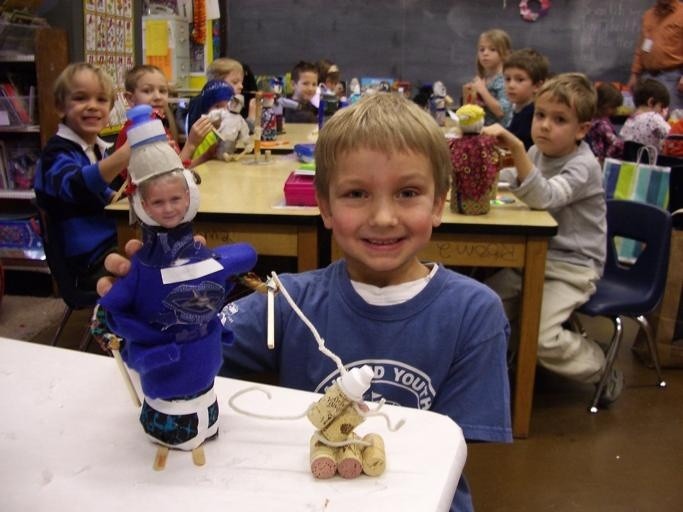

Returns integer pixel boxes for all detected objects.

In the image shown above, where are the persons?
[483,71,625,405]
[622,0,683,110]
[317,59,346,102]
[188,57,257,141]
[95,105,258,473]
[260,76,301,133]
[284,60,334,123]
[261,93,277,141]
[114,64,214,195]
[585,82,625,161]
[32,62,133,309]
[201,94,256,162]
[194,79,250,162]
[447,104,506,215]
[618,78,672,156]
[502,46,552,152]
[472,29,515,129]
[430,80,453,126]
[349,77,361,102]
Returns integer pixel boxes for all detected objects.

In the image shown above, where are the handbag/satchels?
[631,229,683,369]
[602,145,671,265]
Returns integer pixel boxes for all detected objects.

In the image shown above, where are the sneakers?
[593,344,622,405]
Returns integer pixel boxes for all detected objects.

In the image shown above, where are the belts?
[643,64,680,77]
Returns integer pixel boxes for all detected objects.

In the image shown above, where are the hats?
[200,80,236,117]
